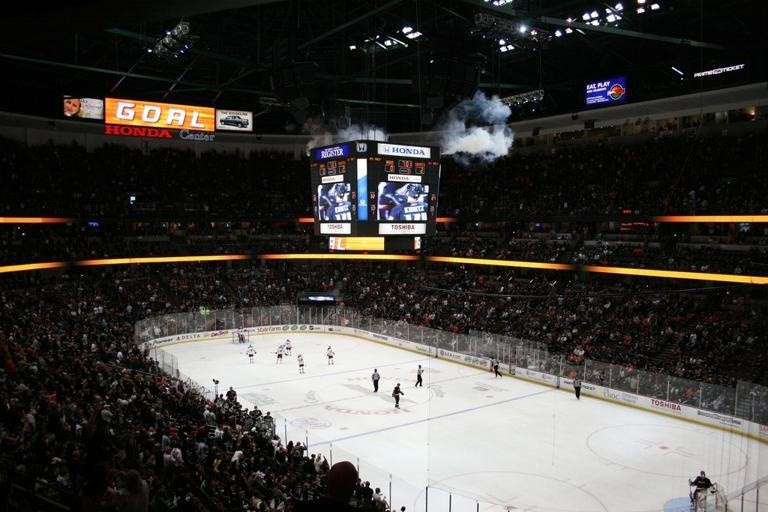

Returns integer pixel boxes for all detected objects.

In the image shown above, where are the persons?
[0,368,412,512]
[687,470,713,510]
[0,122,531,368]
[488,356,503,378]
[530,101,767,428]
[414,365,424,387]
